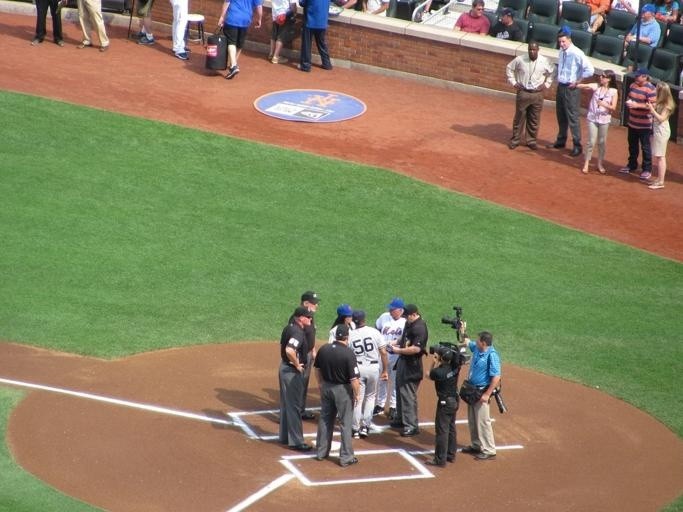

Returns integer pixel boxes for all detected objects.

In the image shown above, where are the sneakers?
[30,31,191,59]
[226,65,239,78]
[620,167,664,189]
[268,55,331,71]
[582,166,606,174]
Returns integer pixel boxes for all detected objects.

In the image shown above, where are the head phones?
[438,350,451,361]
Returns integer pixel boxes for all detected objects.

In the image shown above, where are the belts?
[357,361,378,364]
[523,88,543,93]
[559,82,570,87]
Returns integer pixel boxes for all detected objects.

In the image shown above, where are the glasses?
[602,74,610,78]
[477,8,485,11]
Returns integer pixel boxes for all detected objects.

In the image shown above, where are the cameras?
[491,388,509,414]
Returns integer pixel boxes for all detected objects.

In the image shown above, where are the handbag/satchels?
[459,380,482,405]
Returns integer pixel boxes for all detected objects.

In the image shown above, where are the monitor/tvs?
[441,306,463,329]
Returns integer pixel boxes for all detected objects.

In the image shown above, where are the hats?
[386,298,405,308]
[295,307,313,318]
[500,7,514,16]
[400,304,417,317]
[434,346,453,359]
[337,304,353,315]
[631,67,649,75]
[352,310,365,320]
[336,324,348,335]
[302,291,321,304]
[642,4,656,12]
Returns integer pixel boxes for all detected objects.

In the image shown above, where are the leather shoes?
[510,144,582,156]
[447,457,455,462]
[476,453,496,459]
[462,447,480,453]
[281,405,418,465]
[426,459,445,466]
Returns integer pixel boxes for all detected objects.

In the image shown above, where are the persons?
[168,0,192,59]
[460,320,501,460]
[505,42,555,151]
[77,0,110,52]
[425,348,460,467]
[313,298,429,468]
[130,1,156,44]
[269,0,296,66]
[30,0,67,47]
[647,83,675,190]
[576,0,682,57]
[218,0,263,79]
[453,0,490,36]
[489,8,523,41]
[619,69,656,178]
[579,73,617,175]
[547,35,594,154]
[297,0,332,71]
[331,0,449,21]
[277,291,322,451]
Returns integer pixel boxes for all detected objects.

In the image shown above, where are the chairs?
[388,0,683,87]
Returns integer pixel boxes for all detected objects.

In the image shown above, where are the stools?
[183,14,206,46]
